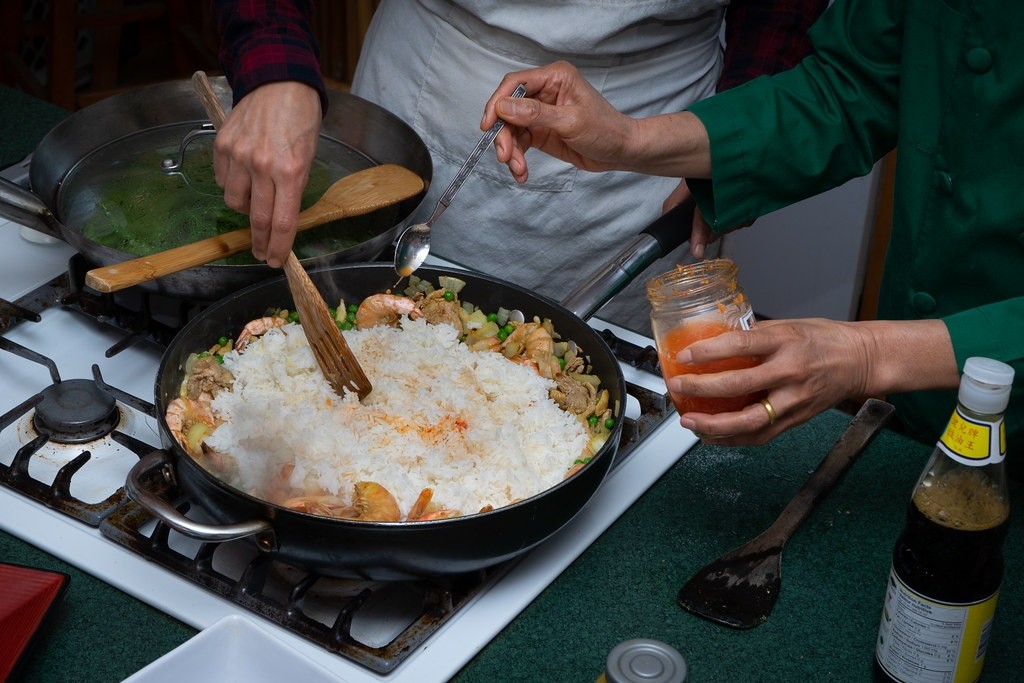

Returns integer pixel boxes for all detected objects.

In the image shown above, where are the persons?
[482,2,1023,453]
[205,1,744,338]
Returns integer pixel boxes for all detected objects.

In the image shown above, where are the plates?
[114,614,345,683]
[0,562,72,683]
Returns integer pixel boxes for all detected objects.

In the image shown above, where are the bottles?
[645,256,764,442]
[872,355,1015,683]
[593,638,689,683]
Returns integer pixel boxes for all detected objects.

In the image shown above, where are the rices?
[202,324,586,515]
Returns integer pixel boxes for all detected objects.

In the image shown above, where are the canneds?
[646,261,762,442]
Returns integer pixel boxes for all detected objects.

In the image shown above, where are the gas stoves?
[0,180,703,683]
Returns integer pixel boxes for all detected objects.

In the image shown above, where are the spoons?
[391,81,527,277]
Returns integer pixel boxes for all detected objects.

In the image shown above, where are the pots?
[125,197,697,573]
[0,71,435,301]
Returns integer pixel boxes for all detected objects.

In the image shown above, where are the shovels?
[676,396,895,630]
[85,161,425,290]
[189,68,374,408]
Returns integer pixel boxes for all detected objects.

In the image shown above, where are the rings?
[761,399,776,424]
